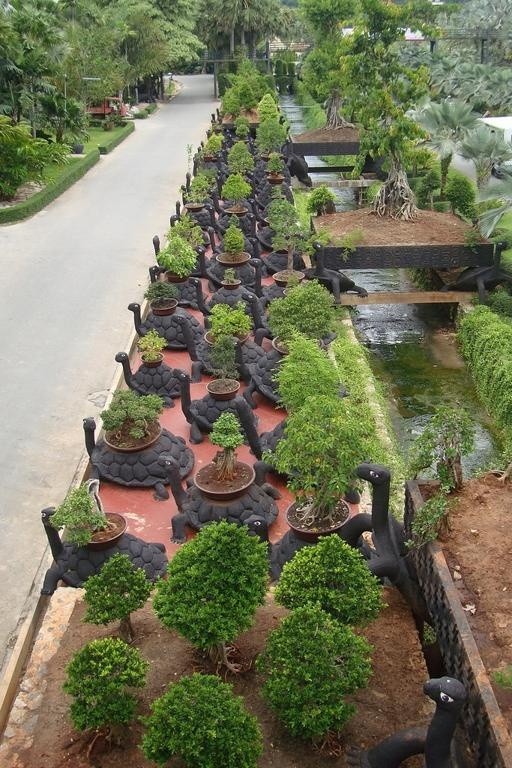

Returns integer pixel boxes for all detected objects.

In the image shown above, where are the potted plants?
[263,393,373,544]
[193,410,256,500]
[145,282,178,315]
[181,173,216,212]
[203,304,247,348]
[264,198,317,286]
[199,91,289,185]
[48,484,127,549]
[99,387,164,454]
[155,237,199,283]
[221,267,242,290]
[215,225,252,266]
[205,333,240,401]
[136,329,168,368]
[265,277,335,356]
[219,174,253,217]
